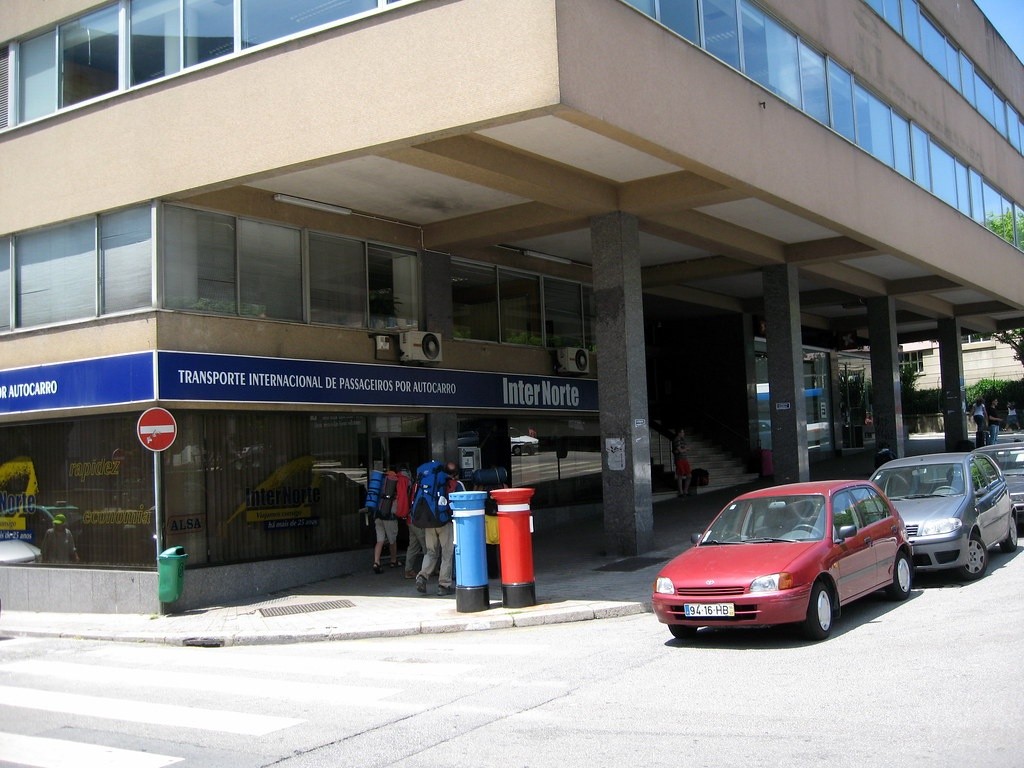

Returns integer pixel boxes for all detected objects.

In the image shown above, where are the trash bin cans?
[875,452,895,469]
[158,546,188,602]
[976,431,990,449]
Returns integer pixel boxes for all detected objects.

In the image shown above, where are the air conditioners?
[556,347,590,374]
[399,331,443,363]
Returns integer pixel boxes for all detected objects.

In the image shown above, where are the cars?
[651,480,918,641]
[508,427,540,456]
[0,503,159,570]
[969,442,1024,523]
[857,452,1018,580]
[310,470,367,504]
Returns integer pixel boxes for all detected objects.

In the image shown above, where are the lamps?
[273,193,352,216]
[523,250,573,265]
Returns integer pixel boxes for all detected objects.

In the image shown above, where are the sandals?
[373,563,384,574]
[390,561,403,568]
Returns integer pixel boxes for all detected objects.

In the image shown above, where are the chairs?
[892,471,916,496]
[756,507,787,538]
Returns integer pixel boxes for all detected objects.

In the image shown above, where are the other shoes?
[405,571,418,578]
[437,585,453,595]
[416,576,426,592]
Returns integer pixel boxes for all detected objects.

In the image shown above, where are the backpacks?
[412,460,456,529]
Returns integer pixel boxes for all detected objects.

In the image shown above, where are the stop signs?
[136,408,177,451]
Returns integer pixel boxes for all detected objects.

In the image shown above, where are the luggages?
[976,432,991,447]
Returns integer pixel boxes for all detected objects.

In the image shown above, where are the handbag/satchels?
[955,441,975,452]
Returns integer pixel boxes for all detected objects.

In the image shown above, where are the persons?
[1004,400,1020,431]
[41,514,79,563]
[672,428,691,495]
[528,425,537,440]
[946,466,962,491]
[794,502,839,541]
[366,462,510,596]
[971,398,987,432]
[988,397,1002,445]
